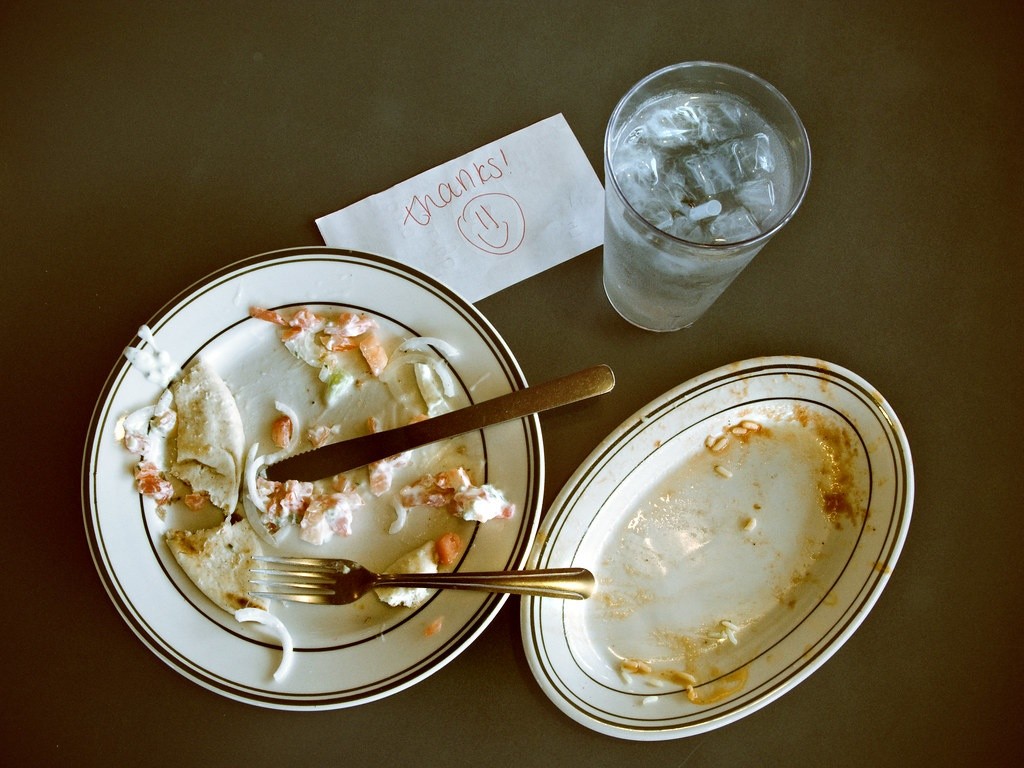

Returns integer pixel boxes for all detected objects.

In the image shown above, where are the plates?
[520,355,915,742]
[80,245,547,713]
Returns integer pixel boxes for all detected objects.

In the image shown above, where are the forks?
[247,555,596,606]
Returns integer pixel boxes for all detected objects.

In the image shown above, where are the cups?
[604,60,813,333]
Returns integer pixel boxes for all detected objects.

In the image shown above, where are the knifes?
[259,365,617,484]
